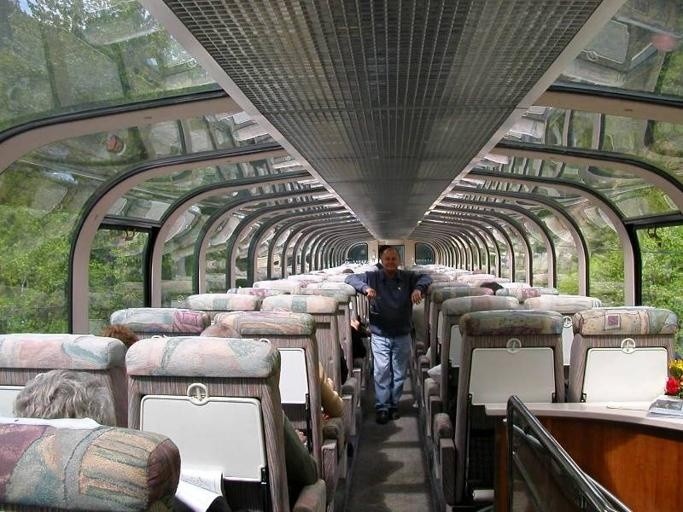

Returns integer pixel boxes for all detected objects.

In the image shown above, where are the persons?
[16,367,118,430]
[343,269,354,273]
[345,247,432,425]
[480,282,504,295]
[199,323,319,511]
[318,361,344,420]
[101,324,140,348]
[349,319,366,360]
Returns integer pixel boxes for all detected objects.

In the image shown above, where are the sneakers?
[376,410,389,425]
[388,408,400,420]
